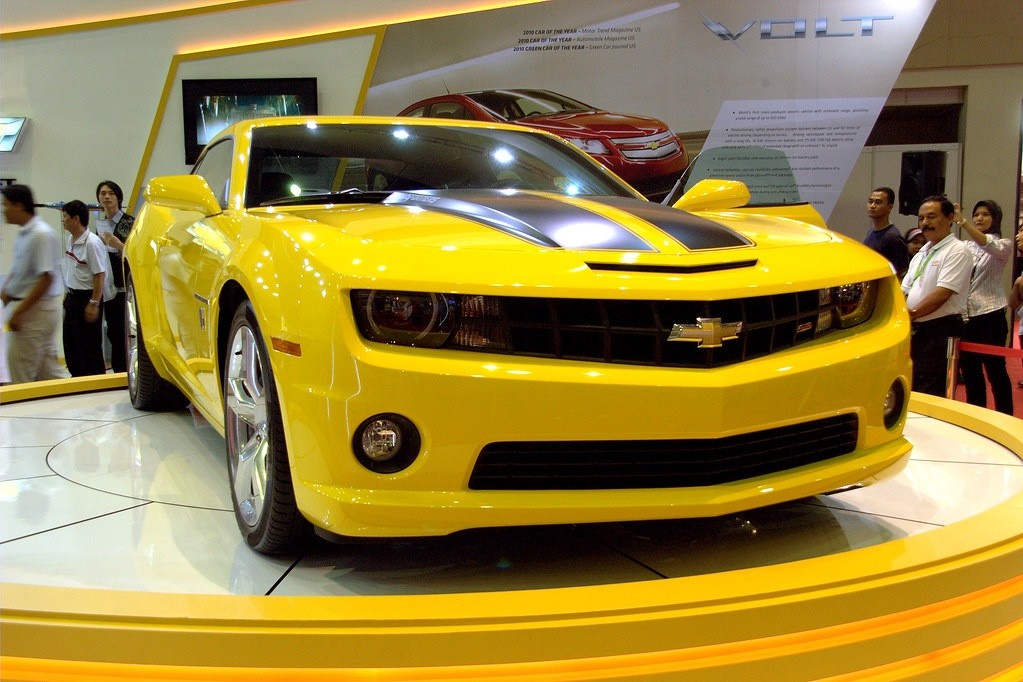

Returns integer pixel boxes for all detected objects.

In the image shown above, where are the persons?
[950,200,1014,415]
[0,185,72,383]
[903,228,928,254]
[96,180,136,371]
[900,196,973,398]
[61,199,107,376]
[1008,223,1023,386]
[862,187,909,278]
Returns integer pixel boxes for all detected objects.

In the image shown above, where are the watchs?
[911,309,917,317]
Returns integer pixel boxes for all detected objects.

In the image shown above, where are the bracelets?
[89,300,100,306]
[957,219,967,226]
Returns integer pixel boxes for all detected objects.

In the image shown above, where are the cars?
[120,115,914,557]
[363,88,687,208]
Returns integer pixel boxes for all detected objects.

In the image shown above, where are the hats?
[906,229,923,240]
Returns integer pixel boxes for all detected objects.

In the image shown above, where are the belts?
[9,297,21,301]
